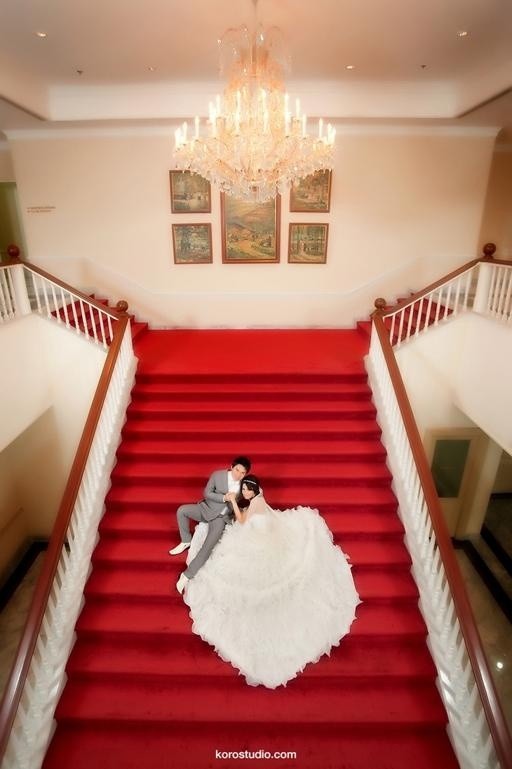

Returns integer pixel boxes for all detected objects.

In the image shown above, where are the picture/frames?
[168,168,214,215]
[170,221,216,266]
[218,176,283,266]
[288,165,334,213]
[287,219,330,264]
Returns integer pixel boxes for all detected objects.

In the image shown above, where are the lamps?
[166,0,339,206]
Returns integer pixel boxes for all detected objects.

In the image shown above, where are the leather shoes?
[176,573,188,594]
[169,543,191,555]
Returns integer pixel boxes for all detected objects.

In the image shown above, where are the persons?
[169,456,250,595]
[183,475,364,690]
